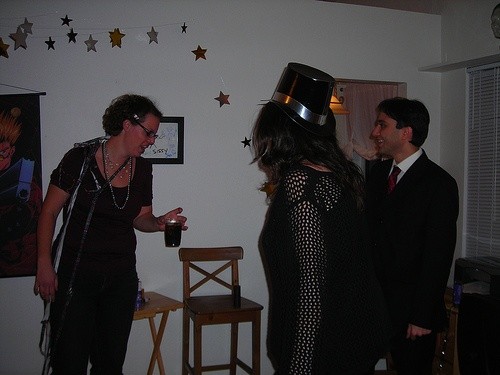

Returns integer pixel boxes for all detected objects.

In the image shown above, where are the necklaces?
[102,139,134,210]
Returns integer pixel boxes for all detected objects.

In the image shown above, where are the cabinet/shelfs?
[433,293,461,375]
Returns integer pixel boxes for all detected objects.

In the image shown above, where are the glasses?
[132,117,159,139]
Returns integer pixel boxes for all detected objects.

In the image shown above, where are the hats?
[259,62,336,137]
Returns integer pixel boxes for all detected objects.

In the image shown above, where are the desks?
[135,292,184,375]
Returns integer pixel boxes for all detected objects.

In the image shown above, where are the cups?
[164,218,181,247]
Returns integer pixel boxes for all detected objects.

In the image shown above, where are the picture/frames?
[140,118,184,164]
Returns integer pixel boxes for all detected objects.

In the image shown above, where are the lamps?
[330,96,350,114]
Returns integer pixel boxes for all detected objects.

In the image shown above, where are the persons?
[364,97,459,375]
[249,62,392,375]
[34,94,189,375]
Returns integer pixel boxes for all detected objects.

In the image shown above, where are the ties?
[387,166,402,193]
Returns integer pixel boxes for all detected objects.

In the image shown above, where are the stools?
[179,246,264,375]
